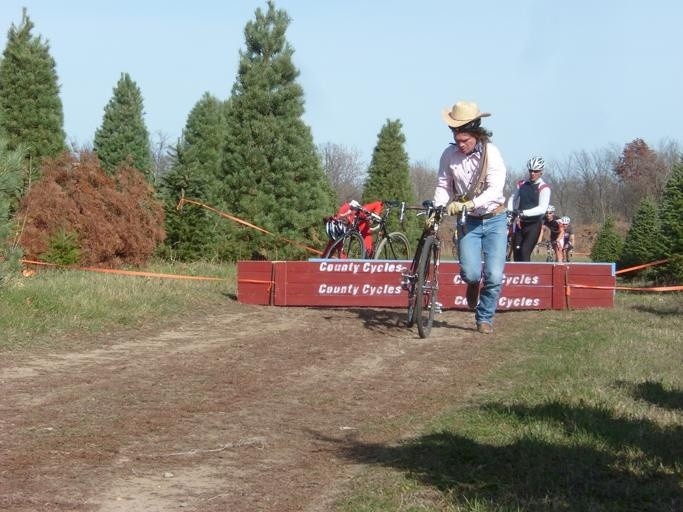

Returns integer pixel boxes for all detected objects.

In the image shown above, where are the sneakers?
[465,278,480,308]
[475,324,494,335]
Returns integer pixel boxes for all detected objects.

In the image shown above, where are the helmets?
[325,219,347,242]
[526,156,545,172]
[545,205,571,225]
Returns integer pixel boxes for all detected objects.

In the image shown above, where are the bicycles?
[321,197,412,261]
[393,194,470,340]
[449,198,573,265]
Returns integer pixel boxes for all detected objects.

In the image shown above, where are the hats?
[440,99,493,129]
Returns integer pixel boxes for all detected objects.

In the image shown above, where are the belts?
[464,204,504,220]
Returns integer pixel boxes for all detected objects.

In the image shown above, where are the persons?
[507,178,524,233]
[537,205,565,263]
[320,200,384,260]
[560,214,576,260]
[417,116,513,334]
[511,155,553,261]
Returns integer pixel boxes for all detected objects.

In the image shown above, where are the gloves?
[446,201,464,217]
